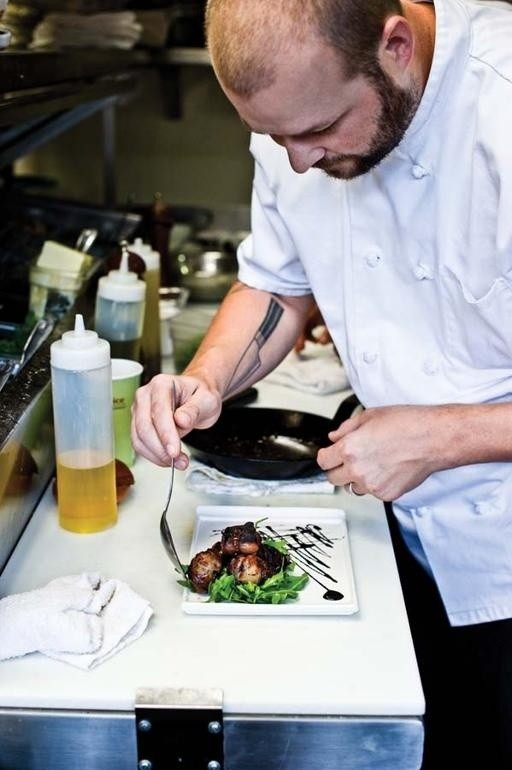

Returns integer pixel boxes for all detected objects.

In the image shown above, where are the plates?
[180,503,359,618]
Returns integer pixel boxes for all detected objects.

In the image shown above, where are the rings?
[348,480,367,498]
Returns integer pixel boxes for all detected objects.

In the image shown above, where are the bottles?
[50,236,164,534]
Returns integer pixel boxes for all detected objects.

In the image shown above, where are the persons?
[128,0,511,768]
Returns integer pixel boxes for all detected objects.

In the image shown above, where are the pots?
[177,394,361,481]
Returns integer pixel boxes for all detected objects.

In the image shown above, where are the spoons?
[160,378,190,582]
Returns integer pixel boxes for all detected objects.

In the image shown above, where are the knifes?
[220,298,284,400]
